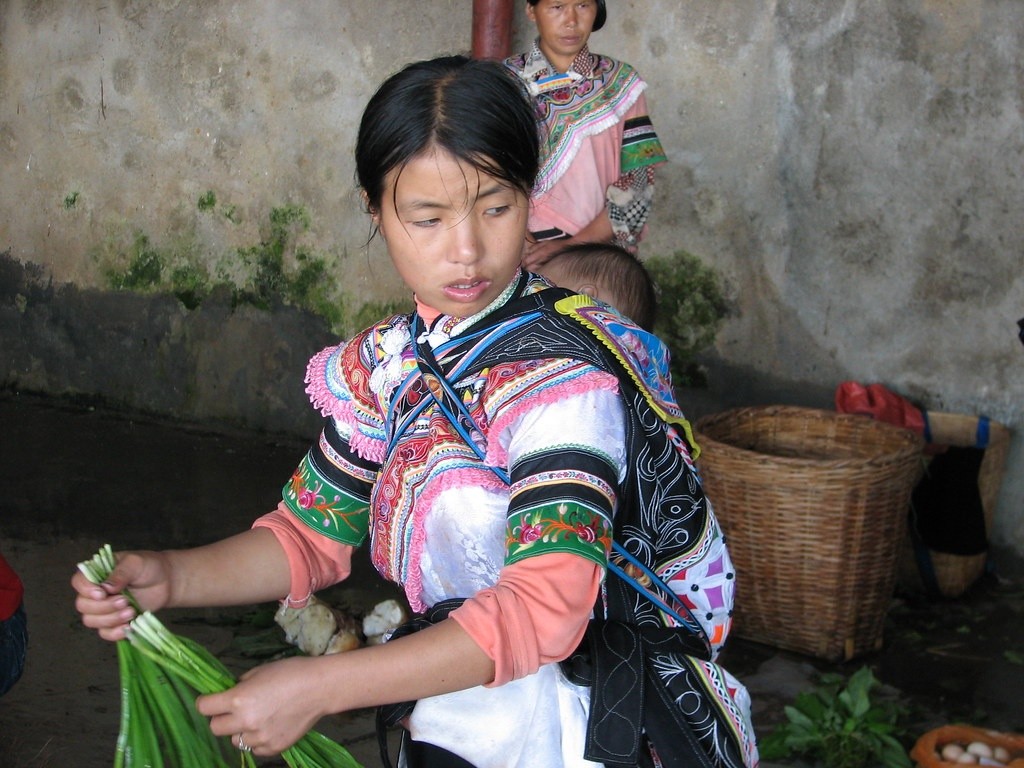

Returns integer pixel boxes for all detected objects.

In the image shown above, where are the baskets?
[692,405,925,660]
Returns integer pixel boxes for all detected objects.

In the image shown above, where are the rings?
[238,733,251,752]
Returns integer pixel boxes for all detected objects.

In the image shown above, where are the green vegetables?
[76,542,367,768]
[754,664,924,768]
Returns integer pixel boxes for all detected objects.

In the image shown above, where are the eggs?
[935,740,1011,767]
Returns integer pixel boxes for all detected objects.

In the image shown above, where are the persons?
[72,56,760,768]
[535,242,656,334]
[502,0,669,274]
